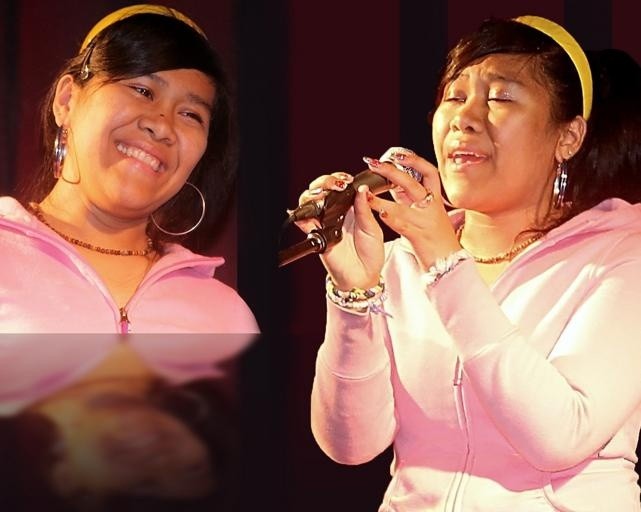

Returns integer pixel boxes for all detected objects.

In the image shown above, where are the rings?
[418,192,436,210]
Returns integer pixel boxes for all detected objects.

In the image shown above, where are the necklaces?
[454,224,544,263]
[25,203,156,259]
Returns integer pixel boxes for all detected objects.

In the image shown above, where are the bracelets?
[325,275,398,319]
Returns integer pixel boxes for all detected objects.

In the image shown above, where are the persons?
[285,15,641,511]
[1,1,263,417]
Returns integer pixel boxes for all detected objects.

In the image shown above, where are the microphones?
[288,161,423,222]
